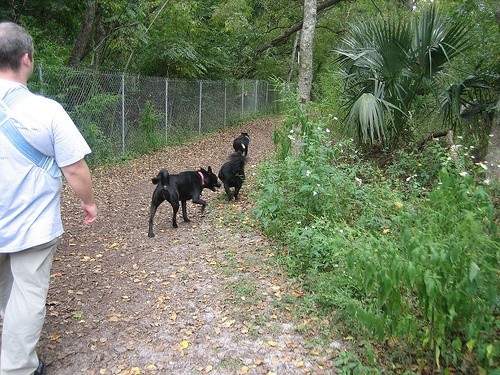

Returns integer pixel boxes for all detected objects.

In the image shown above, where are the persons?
[0,22,97,375]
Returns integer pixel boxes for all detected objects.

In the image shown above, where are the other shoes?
[34,358,44,375]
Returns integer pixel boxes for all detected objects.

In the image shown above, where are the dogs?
[148,166,221,238]
[233,131,251,157]
[218,152,246,201]
